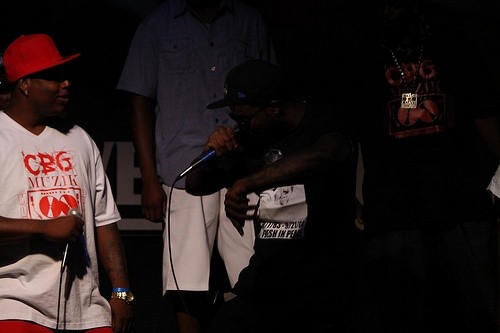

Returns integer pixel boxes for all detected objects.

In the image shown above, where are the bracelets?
[112,287,132,293]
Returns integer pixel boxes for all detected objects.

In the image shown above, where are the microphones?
[61,209,82,273]
[176,148,216,181]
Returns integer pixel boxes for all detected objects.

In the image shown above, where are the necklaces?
[384,34,425,106]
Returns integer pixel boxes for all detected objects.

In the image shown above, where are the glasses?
[229,107,262,127]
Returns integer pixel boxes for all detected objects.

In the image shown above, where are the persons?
[0,50,13,114]
[179,59,400,333]
[347,0,500,332]
[117,0,285,333]
[0,33,135,333]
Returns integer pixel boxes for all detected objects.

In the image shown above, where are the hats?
[2,34,81,84]
[205,58,287,108]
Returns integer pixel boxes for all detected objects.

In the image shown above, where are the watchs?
[110,291,134,303]
